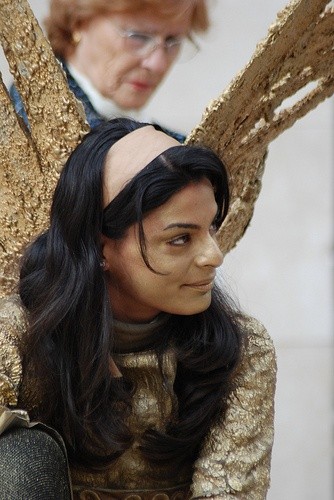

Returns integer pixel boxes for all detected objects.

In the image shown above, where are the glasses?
[107,20,200,63]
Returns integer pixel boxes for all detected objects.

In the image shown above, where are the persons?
[0,117,277,500]
[8,0,210,134]
